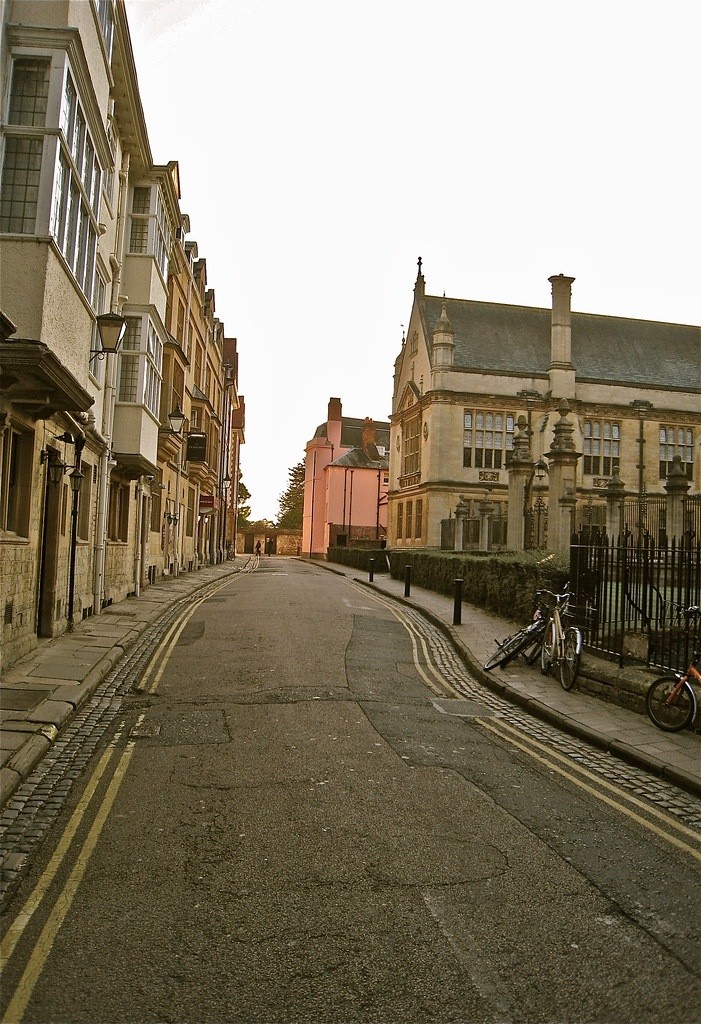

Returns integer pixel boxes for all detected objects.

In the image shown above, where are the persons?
[255,539,261,556]
[267,537,273,557]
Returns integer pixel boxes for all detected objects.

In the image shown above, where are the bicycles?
[226,539,235,561]
[644,600,701,731]
[484,592,550,671]
[535,588,582,691]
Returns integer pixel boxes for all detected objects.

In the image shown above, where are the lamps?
[159,402,187,434]
[40,450,66,487]
[215,475,232,489]
[171,513,179,526]
[89,311,128,364]
[164,512,173,526]
[63,464,85,495]
